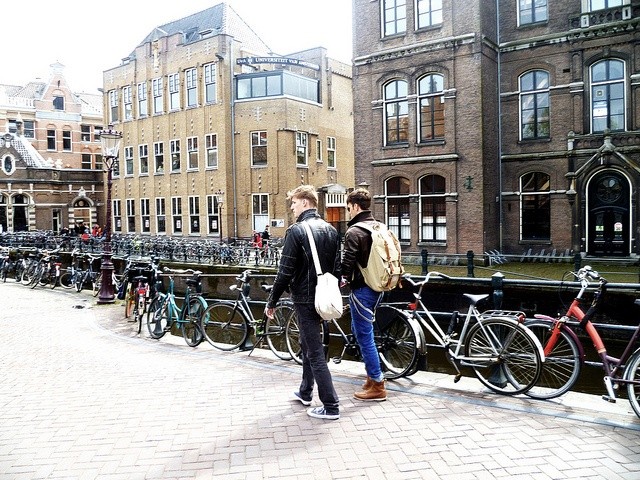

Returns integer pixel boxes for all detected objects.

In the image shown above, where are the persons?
[340,188,387,401]
[60,222,107,240]
[263,185,342,420]
[251,225,272,249]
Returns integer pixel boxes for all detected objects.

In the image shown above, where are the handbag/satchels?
[315,272,343,321]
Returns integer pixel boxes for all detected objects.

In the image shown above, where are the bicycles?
[216,237,281,268]
[124,261,149,335]
[112,232,216,268]
[284,279,421,380]
[501,264,640,425]
[375,270,546,396]
[0,227,102,298]
[199,268,303,361]
[147,274,210,348]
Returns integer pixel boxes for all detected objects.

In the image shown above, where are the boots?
[361,376,371,390]
[353,380,387,401]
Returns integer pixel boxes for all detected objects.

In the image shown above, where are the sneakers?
[307,406,339,420]
[293,391,312,406]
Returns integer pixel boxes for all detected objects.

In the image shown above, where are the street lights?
[215,189,225,245]
[96,123,124,305]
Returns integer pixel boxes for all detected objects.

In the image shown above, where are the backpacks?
[348,222,405,292]
